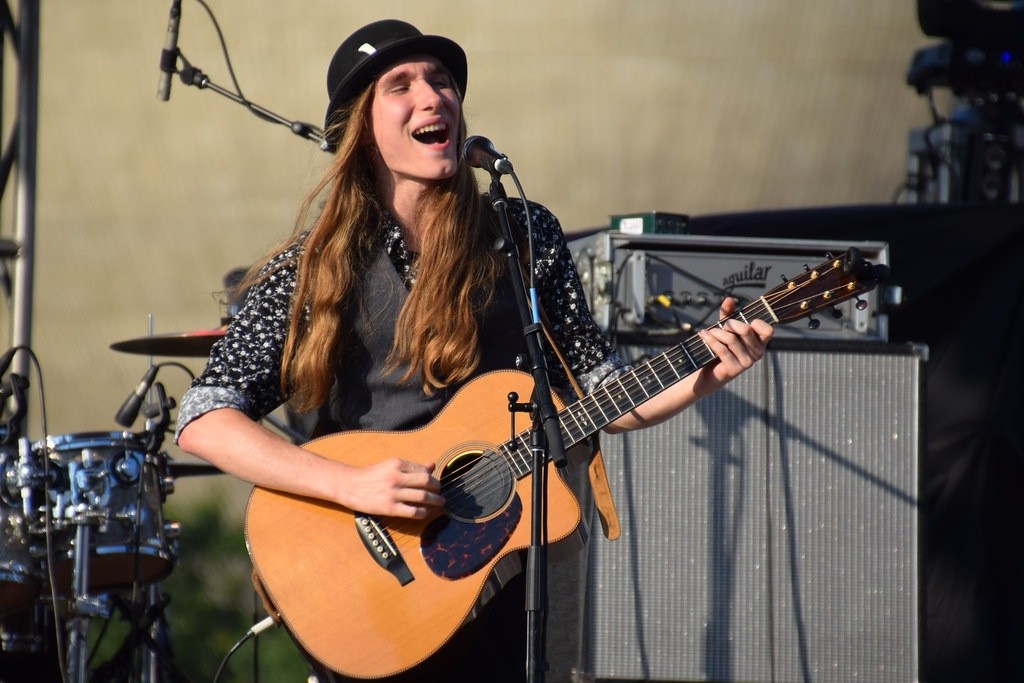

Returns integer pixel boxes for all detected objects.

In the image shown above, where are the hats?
[324,19,468,153]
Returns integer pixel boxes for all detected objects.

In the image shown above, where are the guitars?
[243,247,876,683]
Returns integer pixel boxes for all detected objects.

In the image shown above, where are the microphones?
[156,0,182,101]
[114,365,159,428]
[462,136,513,174]
[0,347,17,381]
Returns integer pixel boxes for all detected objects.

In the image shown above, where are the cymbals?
[110,323,226,357]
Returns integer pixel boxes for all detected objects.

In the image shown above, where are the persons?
[173,18,775,683]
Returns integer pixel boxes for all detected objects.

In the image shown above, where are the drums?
[0,430,177,683]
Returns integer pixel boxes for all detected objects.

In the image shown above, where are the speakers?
[541,330,930,683]
